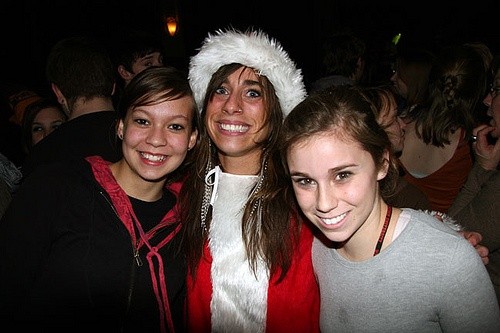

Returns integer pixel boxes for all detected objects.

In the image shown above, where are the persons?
[176,23,488,333]
[0,32,500,304]
[0,66,210,333]
[277,87,500,333]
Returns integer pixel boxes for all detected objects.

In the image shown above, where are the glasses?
[490,84,500,98]
